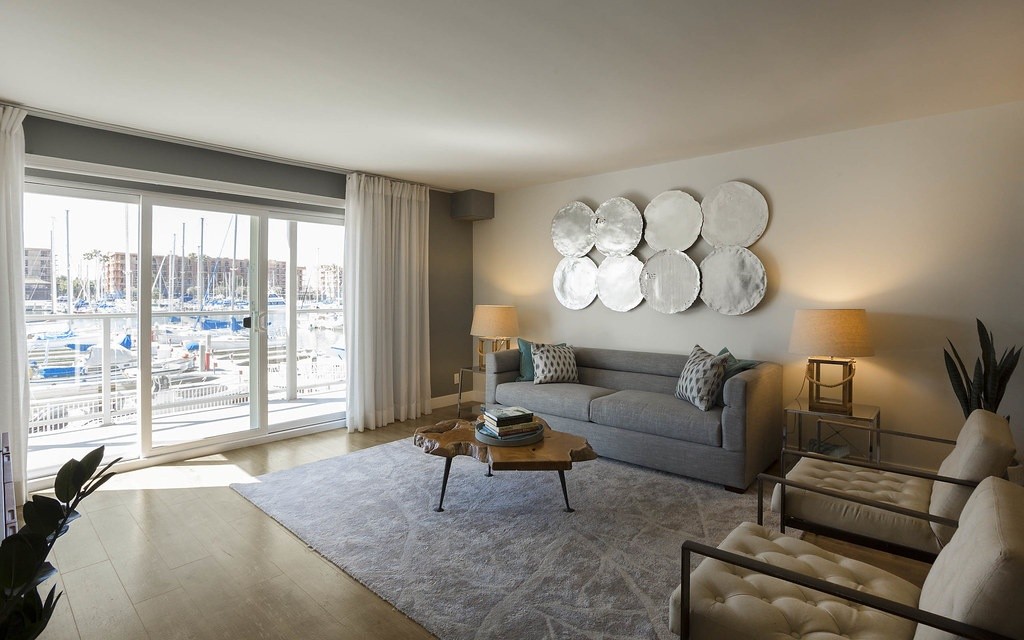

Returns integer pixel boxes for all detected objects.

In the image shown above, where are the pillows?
[528,340,579,384]
[675,344,727,411]
[714,348,757,407]
[515,337,567,382]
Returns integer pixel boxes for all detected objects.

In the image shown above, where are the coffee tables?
[413,419,598,515]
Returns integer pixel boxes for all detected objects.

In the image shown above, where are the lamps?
[470,305,520,369]
[787,308,875,413]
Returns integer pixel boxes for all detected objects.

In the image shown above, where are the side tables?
[457,365,486,417]
[784,395,881,463]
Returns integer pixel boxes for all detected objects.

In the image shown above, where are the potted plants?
[942,317,1024,488]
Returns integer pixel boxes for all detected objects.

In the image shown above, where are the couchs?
[484,345,784,497]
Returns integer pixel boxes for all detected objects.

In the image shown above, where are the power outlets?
[454,373,460,384]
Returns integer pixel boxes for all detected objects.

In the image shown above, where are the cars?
[184,292,193,302]
[57,295,68,302]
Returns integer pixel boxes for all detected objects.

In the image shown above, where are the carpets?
[229,438,808,640]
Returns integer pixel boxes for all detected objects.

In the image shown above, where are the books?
[478,405,544,440]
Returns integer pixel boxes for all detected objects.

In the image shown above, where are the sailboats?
[25,192,346,421]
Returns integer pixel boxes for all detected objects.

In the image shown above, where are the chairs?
[668,472,1024,640]
[769,408,1016,588]
